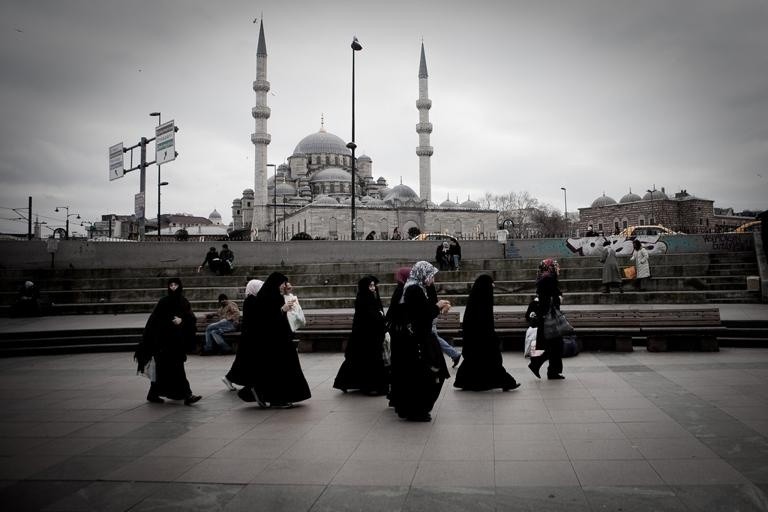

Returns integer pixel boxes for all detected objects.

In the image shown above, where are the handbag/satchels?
[624,266,636,279]
[543,311,574,339]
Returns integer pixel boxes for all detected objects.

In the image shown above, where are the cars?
[617,223,687,240]
[413,232,458,240]
[733,220,759,234]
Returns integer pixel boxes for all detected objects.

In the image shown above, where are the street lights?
[345,35,364,238]
[561,187,570,236]
[149,111,168,241]
[645,189,658,223]
[54,204,81,239]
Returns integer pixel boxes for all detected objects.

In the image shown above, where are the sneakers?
[452,352,461,368]
[547,372,565,380]
[528,361,541,379]
[221,375,292,408]
[503,383,521,391]
[184,395,202,406]
[147,394,164,403]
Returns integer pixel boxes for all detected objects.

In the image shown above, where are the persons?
[628,240,652,291]
[523,258,577,381]
[330,260,522,424]
[435,241,450,271]
[131,277,202,408]
[390,230,401,240]
[218,243,234,270]
[18,279,41,317]
[200,271,313,409]
[447,237,461,272]
[201,246,224,276]
[365,230,377,240]
[596,240,626,294]
